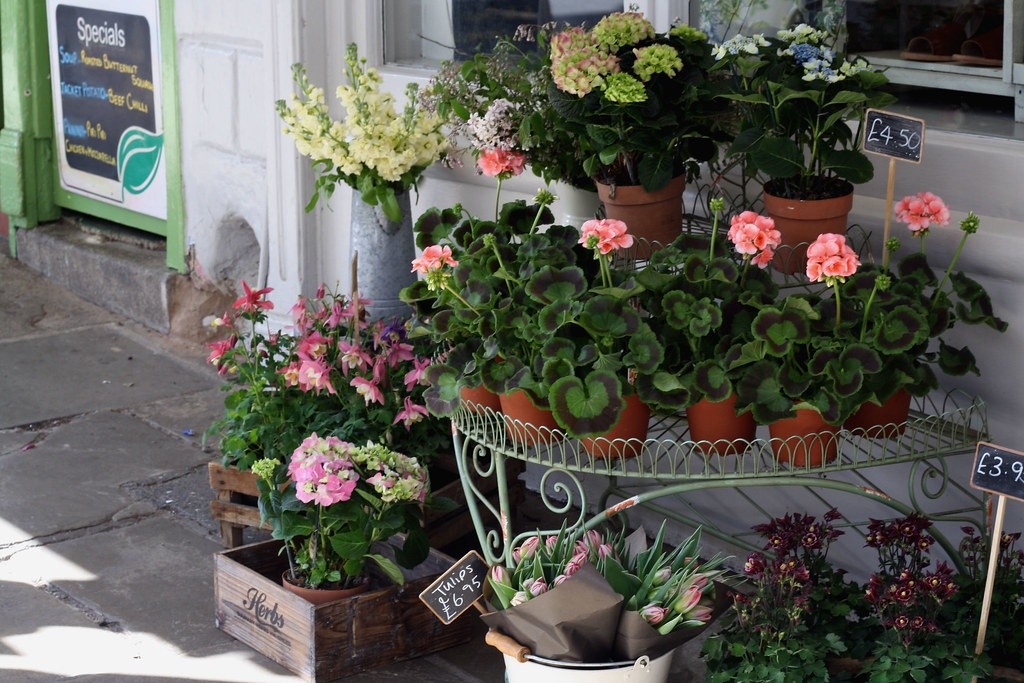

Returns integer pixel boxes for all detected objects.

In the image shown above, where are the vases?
[459,381,501,416]
[281,565,369,605]
[498,388,569,445]
[594,169,686,260]
[581,393,651,456]
[553,179,602,234]
[768,400,841,466]
[826,656,875,682]
[690,389,757,451]
[348,179,420,327]
[764,172,854,275]
[842,378,910,437]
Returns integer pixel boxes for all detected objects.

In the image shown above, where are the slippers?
[900,24,1003,65]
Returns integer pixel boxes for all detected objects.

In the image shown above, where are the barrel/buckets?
[485,628,676,683]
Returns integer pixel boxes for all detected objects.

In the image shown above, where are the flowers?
[251,432,432,590]
[272,41,458,222]
[205,277,453,477]
[396,145,1009,439]
[418,1,895,202]
[489,518,727,646]
[699,508,1024,683]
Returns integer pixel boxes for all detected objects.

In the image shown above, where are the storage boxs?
[213,527,476,683]
